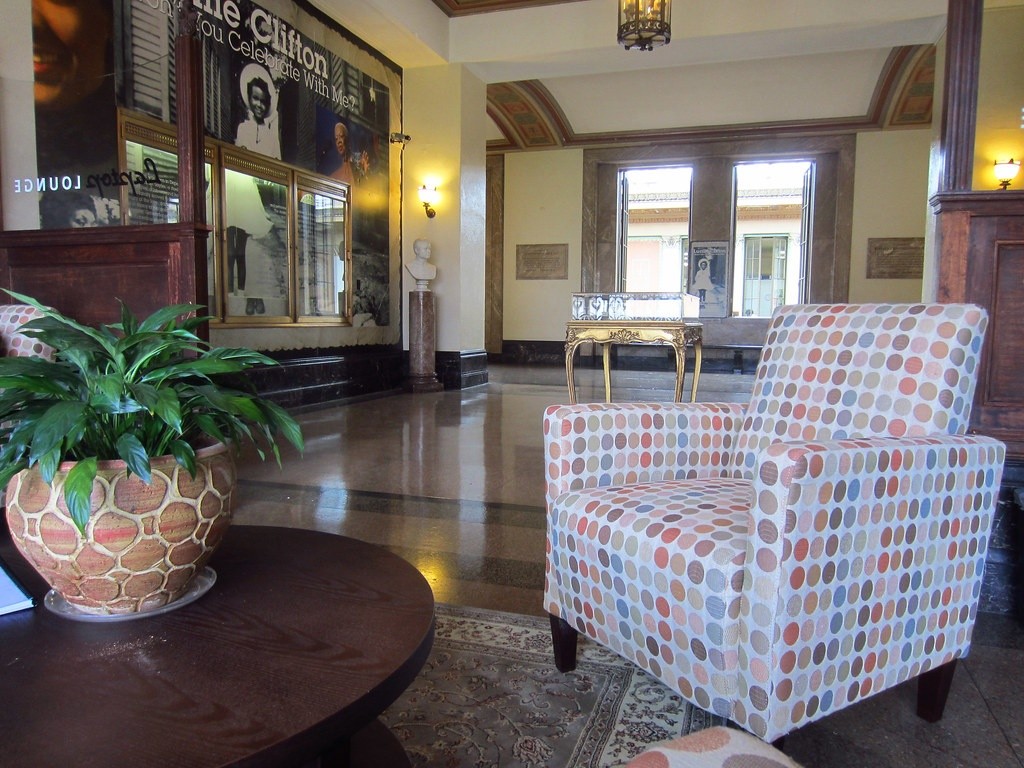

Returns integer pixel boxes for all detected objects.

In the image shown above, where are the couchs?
[545,302,1000,742]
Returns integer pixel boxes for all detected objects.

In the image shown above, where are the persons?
[334,123,376,190]
[695,258,714,308]
[31,0,120,229]
[203,167,274,298]
[233,63,282,160]
[406,239,437,279]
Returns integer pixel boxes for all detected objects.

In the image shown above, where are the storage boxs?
[571,291,701,323]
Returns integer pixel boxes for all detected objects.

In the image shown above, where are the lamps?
[992,159,1022,189]
[419,174,444,219]
[616,0,670,51]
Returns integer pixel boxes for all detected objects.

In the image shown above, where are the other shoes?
[246,298,255,314]
[255,299,265,313]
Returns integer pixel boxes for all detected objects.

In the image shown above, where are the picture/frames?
[687,241,730,318]
[865,237,924,279]
[516,243,569,280]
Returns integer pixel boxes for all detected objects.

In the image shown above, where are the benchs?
[602,341,763,375]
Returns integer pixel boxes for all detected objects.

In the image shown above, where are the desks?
[0,526,459,767]
[562,319,703,402]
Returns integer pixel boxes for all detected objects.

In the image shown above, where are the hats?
[240,63,277,118]
[698,259,709,272]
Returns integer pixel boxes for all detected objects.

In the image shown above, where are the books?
[0,557,38,616]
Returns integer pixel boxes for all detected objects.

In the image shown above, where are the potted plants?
[0,283,308,620]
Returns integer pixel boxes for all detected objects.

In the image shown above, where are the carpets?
[332,602,688,768]
